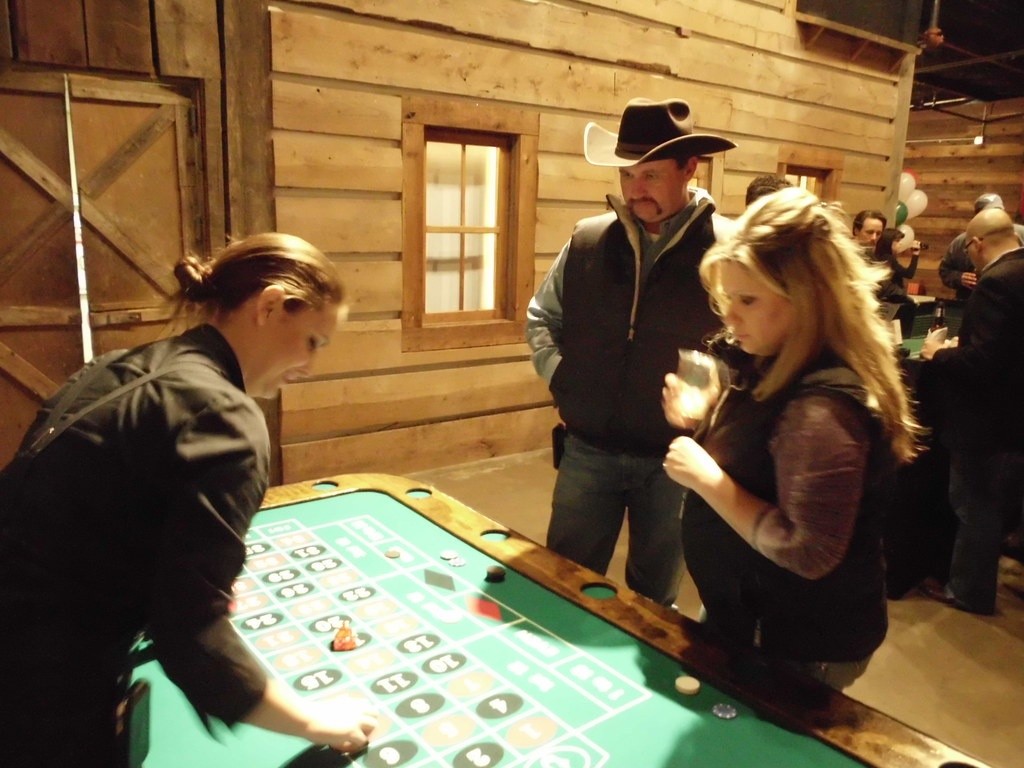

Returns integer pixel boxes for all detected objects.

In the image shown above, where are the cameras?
[920,242,929,250]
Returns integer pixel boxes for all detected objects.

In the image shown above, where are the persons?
[853,193,1024,614]
[746,175,792,206]
[661,186,916,695]
[0,233,375,768]
[525,95,737,610]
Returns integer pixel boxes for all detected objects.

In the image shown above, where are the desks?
[127,472,993,768]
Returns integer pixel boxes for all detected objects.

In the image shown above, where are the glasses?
[962,238,984,254]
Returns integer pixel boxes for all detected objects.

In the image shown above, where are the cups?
[673,348,722,420]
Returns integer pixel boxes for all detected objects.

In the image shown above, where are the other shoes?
[920,578,952,603]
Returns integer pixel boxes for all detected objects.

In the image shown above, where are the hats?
[584,97,738,166]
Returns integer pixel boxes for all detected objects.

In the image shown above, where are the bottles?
[926,300,947,334]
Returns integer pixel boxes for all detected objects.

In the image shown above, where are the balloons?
[894,168,929,254]
